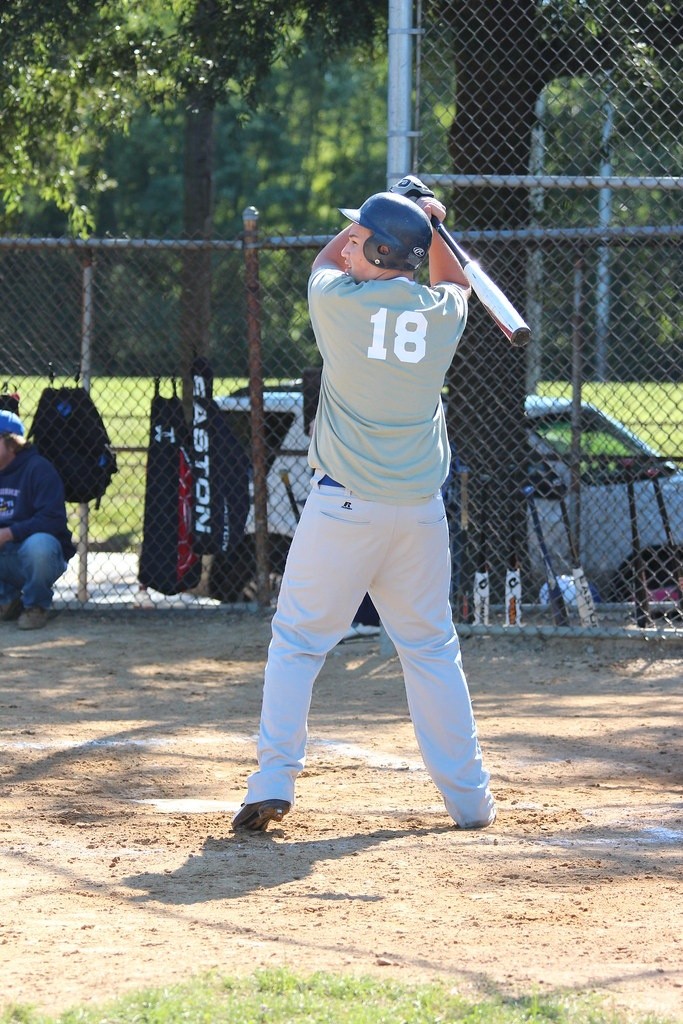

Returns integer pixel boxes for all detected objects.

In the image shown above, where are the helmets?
[338,192,432,270]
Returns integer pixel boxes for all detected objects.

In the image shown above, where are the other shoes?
[232,799,290,831]
[0,599,23,619]
[18,606,46,630]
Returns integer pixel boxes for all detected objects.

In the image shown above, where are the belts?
[311,468,347,487]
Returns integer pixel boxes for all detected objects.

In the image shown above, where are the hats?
[0,411,23,435]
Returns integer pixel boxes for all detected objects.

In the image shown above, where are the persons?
[232,192,496,835]
[0,409,77,632]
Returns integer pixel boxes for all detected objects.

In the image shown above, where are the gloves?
[390,175,434,202]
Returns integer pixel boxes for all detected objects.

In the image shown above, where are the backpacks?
[27,387,117,503]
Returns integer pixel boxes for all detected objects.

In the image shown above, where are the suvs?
[183,380,683,608]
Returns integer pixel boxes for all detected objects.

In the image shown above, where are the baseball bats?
[504,463,520,626]
[623,457,650,629]
[454,465,474,626]
[474,473,493,627]
[279,467,301,523]
[524,485,572,628]
[431,214,531,347]
[648,467,683,599]
[551,477,600,629]
